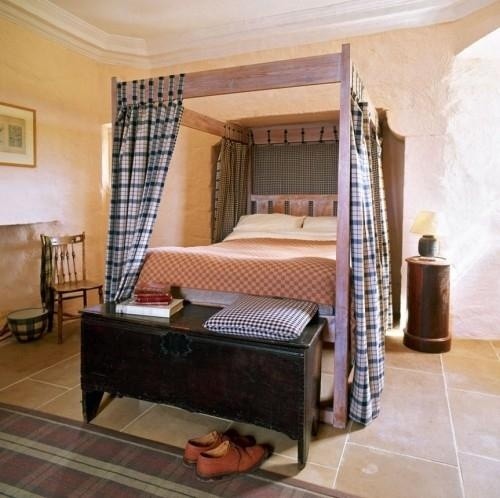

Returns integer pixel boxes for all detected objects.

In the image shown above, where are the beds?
[101,42,405,429]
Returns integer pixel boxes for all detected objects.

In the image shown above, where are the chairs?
[40,231,103,344]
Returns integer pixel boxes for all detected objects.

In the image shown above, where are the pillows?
[202,294,319,341]
[233,213,337,233]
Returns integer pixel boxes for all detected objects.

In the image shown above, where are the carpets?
[0,402,363,498]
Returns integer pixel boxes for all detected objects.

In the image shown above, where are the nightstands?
[403,256,452,353]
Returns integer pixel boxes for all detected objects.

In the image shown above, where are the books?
[115,280,185,319]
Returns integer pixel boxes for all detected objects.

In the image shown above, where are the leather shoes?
[183,430,252,468]
[195,441,269,481]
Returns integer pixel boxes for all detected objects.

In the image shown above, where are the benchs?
[78,297,328,470]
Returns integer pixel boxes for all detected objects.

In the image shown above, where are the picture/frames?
[0,102,36,167]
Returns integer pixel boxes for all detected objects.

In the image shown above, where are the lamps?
[409,210,439,257]
